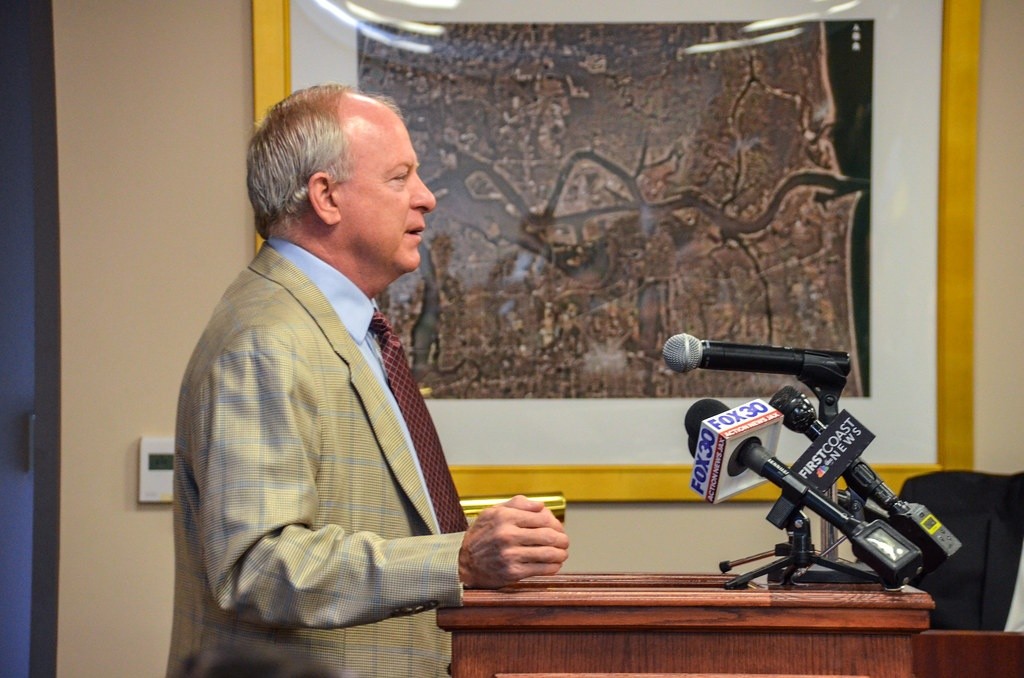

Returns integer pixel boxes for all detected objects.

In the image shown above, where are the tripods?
[718,501,870,590]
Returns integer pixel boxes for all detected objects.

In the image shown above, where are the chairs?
[885,468,1024,640]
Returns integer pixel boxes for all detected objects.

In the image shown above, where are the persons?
[166,83,570,678]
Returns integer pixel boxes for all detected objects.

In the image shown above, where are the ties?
[371,310,469,534]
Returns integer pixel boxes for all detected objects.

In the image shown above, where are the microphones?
[686,386,962,590]
[662,333,852,380]
